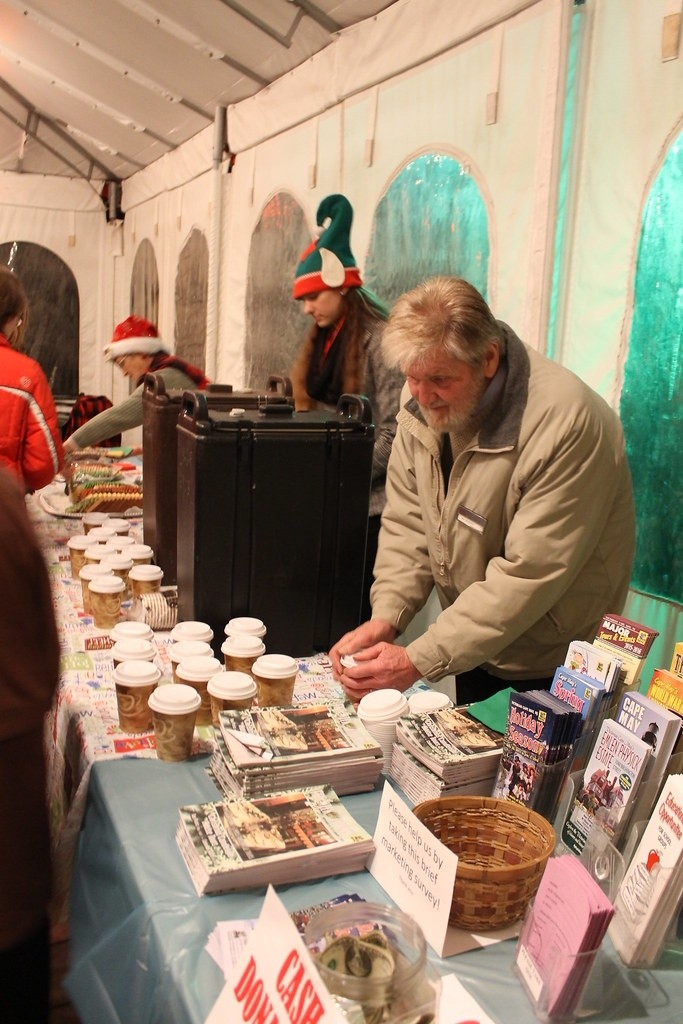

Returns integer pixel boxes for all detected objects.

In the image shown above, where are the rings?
[370,689,373,693]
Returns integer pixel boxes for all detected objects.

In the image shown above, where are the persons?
[288,194,406,623]
[0,264,65,496]
[0,465,63,1023]
[327,276,635,711]
[60,314,213,455]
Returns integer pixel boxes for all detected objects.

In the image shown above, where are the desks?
[24,473,683,1024]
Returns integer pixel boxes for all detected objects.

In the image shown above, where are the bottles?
[300,903,441,1024]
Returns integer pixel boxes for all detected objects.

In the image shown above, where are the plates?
[40,483,86,519]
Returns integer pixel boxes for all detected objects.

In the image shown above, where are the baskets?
[412,796,555,932]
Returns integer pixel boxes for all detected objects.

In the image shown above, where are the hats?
[292,194,363,301]
[102,314,171,362]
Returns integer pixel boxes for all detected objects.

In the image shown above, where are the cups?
[68,510,298,768]
[340,648,373,703]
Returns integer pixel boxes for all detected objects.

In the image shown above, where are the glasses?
[113,354,128,369]
